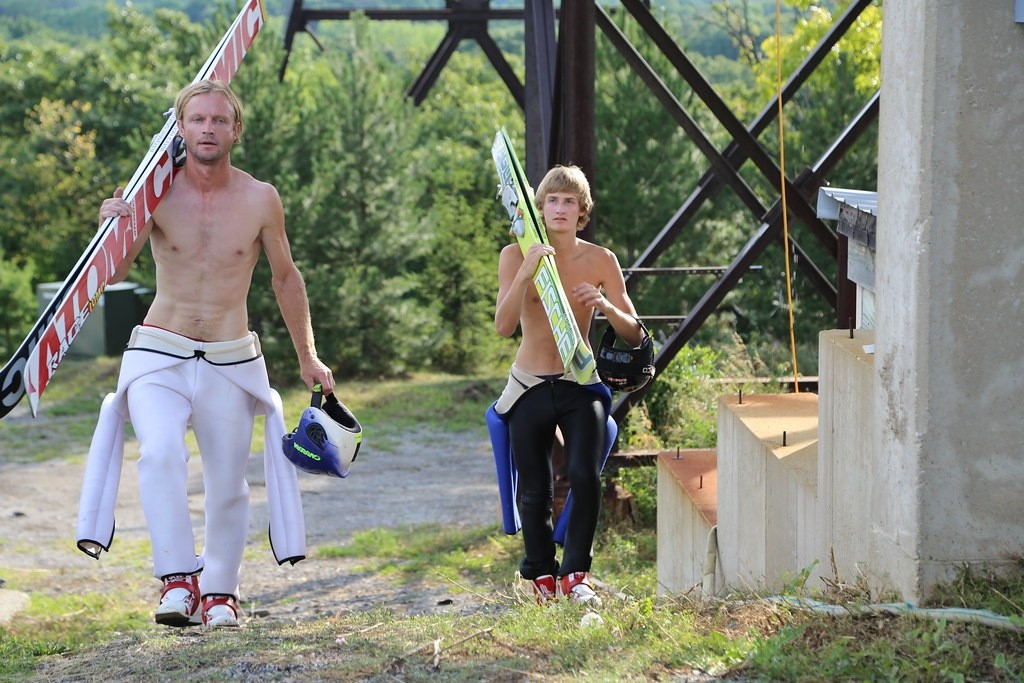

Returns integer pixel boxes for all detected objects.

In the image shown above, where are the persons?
[99,81,335,627]
[495,164,645,608]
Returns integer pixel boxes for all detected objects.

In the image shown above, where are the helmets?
[281,400,363,478]
[595,329,655,393]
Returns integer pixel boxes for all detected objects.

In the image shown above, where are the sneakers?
[561,571,603,613]
[202,593,240,631]
[531,575,563,613]
[155,571,202,626]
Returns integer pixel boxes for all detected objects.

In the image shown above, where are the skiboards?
[0,1,269,420]
[493,127,599,388]
